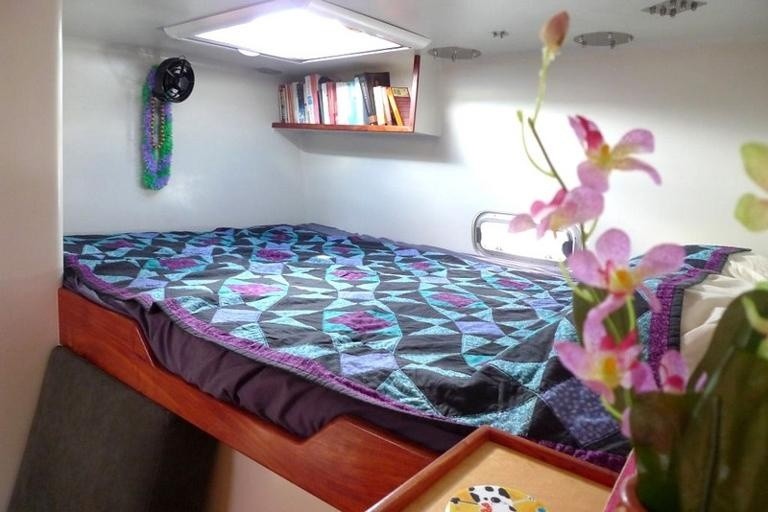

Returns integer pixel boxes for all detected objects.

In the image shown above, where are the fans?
[150,55,194,105]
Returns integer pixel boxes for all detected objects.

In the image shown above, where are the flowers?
[470,10,762,477]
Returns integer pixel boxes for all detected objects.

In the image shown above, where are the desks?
[367,421,619,512]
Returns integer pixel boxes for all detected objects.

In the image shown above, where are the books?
[278,71,410,127]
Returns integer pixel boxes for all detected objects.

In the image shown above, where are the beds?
[62,214,766,512]
